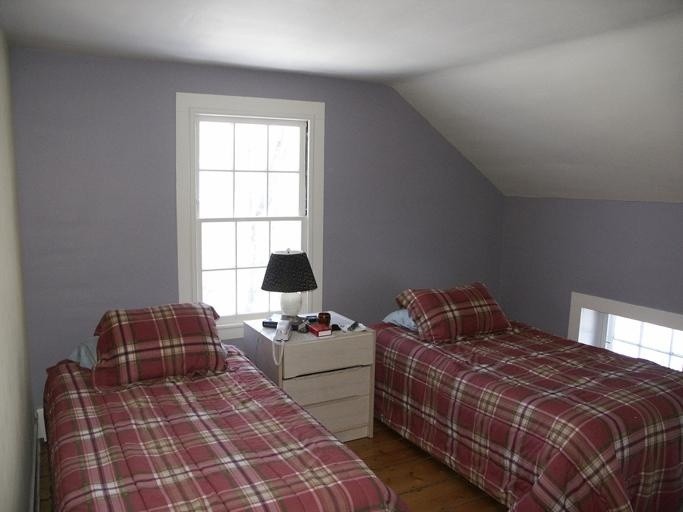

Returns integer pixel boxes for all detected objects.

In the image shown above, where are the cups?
[318,313,330,326]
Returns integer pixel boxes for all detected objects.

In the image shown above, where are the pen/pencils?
[347,321,358,331]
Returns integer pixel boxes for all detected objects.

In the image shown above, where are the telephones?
[274,319,293,341]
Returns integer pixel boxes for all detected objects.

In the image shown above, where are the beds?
[43,344,389,512]
[371,320,683,512]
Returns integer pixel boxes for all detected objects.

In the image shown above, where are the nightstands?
[244,310,377,443]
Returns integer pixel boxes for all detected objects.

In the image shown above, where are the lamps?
[261,251,317,317]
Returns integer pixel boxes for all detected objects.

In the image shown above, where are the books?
[261,313,309,331]
[304,321,332,338]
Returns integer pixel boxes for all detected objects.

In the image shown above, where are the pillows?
[68,335,100,370]
[89,302,226,390]
[397,281,511,345]
[382,309,418,333]
